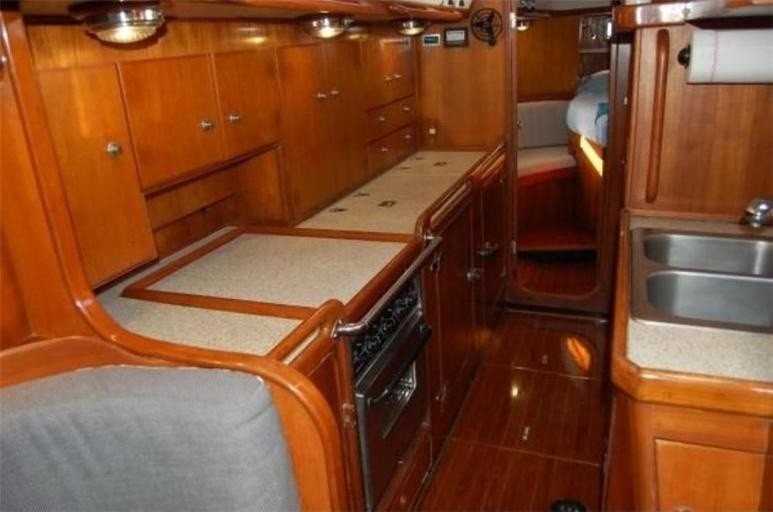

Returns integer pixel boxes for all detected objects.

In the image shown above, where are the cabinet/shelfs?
[611,0,772,221]
[23,21,508,508]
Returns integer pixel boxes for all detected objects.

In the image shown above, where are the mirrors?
[504,0,617,314]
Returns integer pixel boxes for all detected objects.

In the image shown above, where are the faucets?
[743,197,773,231]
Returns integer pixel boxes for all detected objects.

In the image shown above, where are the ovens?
[351,273,433,511]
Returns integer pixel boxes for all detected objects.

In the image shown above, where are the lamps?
[300,11,355,41]
[72,5,165,45]
[390,17,432,37]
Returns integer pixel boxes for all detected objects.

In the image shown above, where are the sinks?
[633,226,772,274]
[631,267,773,328]
